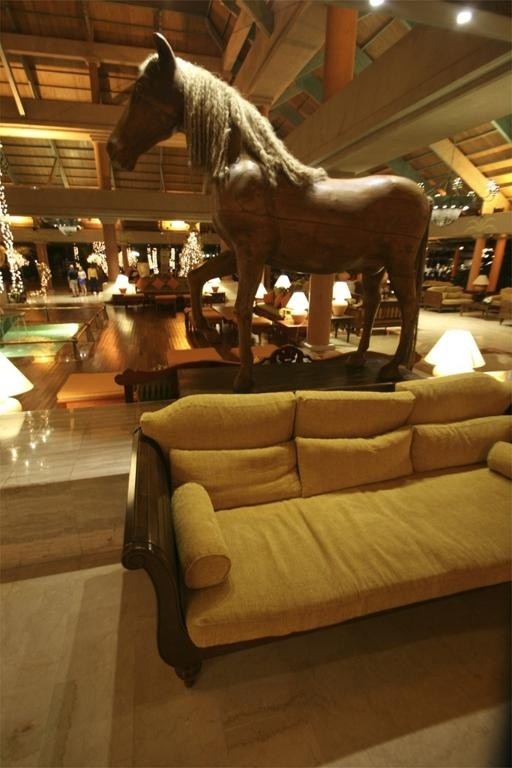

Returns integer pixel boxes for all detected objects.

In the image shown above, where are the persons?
[87,263,99,295]
[76,263,88,295]
[64,264,79,295]
[423,254,470,281]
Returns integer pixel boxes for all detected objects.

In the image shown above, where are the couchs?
[135,273,190,303]
[114,345,423,403]
[254,297,283,321]
[423,286,472,313]
[121,381,512,687]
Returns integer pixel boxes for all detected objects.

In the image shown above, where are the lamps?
[0,353,34,416]
[419,137,500,227]
[423,329,486,376]
[332,281,352,316]
[286,292,309,324]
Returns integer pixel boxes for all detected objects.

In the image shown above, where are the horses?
[104,32,436,394]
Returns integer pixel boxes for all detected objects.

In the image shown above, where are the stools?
[155,295,177,318]
[182,294,191,308]
[124,294,145,312]
[202,294,212,308]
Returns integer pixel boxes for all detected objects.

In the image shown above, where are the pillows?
[292,424,417,498]
[136,277,181,290]
[166,441,302,511]
[410,414,510,475]
[292,387,418,441]
[141,390,297,452]
[264,291,291,308]
[394,373,509,424]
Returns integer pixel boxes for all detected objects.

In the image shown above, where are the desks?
[205,293,225,304]
[183,306,272,345]
[331,313,355,342]
[277,319,308,345]
[57,371,130,407]
[167,343,280,368]
[112,294,124,305]
